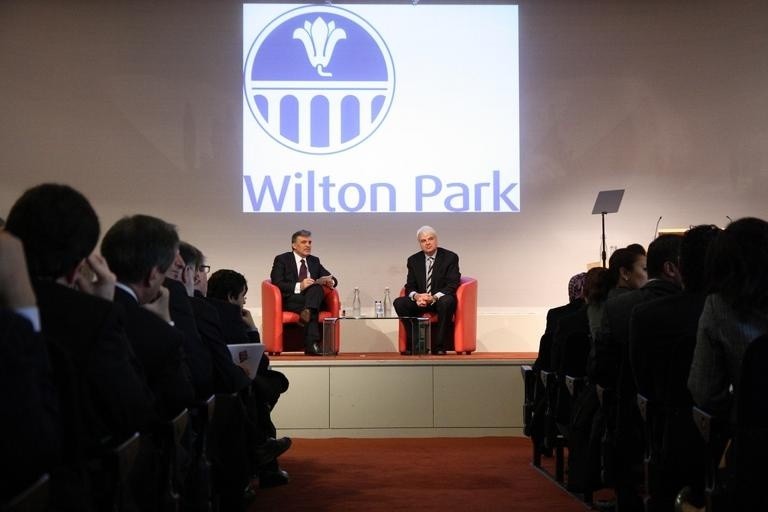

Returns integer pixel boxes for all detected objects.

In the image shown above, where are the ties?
[426,257,435,295]
[298,259,307,281]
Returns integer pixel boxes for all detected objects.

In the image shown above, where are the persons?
[393,226,461,355]
[1,184,292,512]
[533,216,767,511]
[271,230,337,356]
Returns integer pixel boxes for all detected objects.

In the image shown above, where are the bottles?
[384,287,391,317]
[353,288,360,318]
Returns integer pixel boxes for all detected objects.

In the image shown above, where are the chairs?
[0,385,258,511]
[520,363,767,512]
[399,278,477,355]
[260,278,341,357]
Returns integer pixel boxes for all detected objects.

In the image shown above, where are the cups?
[375,300,383,318]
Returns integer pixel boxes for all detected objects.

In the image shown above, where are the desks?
[323,309,430,354]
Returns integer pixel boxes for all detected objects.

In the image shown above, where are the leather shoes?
[263,437,292,458]
[259,467,289,488]
[299,309,310,326]
[305,343,328,355]
[434,349,445,354]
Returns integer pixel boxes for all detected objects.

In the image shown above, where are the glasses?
[200,264,210,274]
